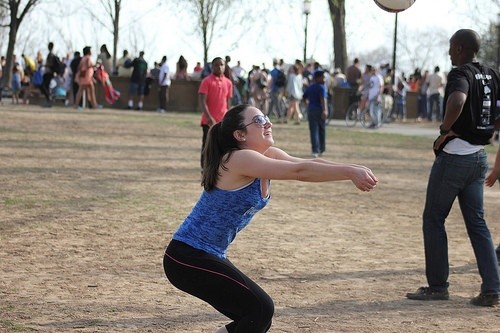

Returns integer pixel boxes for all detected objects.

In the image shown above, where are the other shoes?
[311,152,327,158]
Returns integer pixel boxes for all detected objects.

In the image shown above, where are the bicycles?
[266,91,333,125]
[345,94,375,129]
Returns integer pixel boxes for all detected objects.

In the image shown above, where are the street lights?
[303,0,311,62]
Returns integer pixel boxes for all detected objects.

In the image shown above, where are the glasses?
[239,115,270,130]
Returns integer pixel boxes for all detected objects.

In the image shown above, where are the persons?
[0,41,212,113]
[485,143,500,265]
[223,42,446,129]
[406,29,500,307]
[301,70,329,158]
[163,103,379,333]
[199,57,233,175]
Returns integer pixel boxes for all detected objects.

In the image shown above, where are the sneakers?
[406,286,449,301]
[466,292,500,307]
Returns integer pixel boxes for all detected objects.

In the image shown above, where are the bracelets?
[439,125,449,135]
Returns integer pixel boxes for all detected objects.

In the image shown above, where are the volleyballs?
[374,0,416,14]
